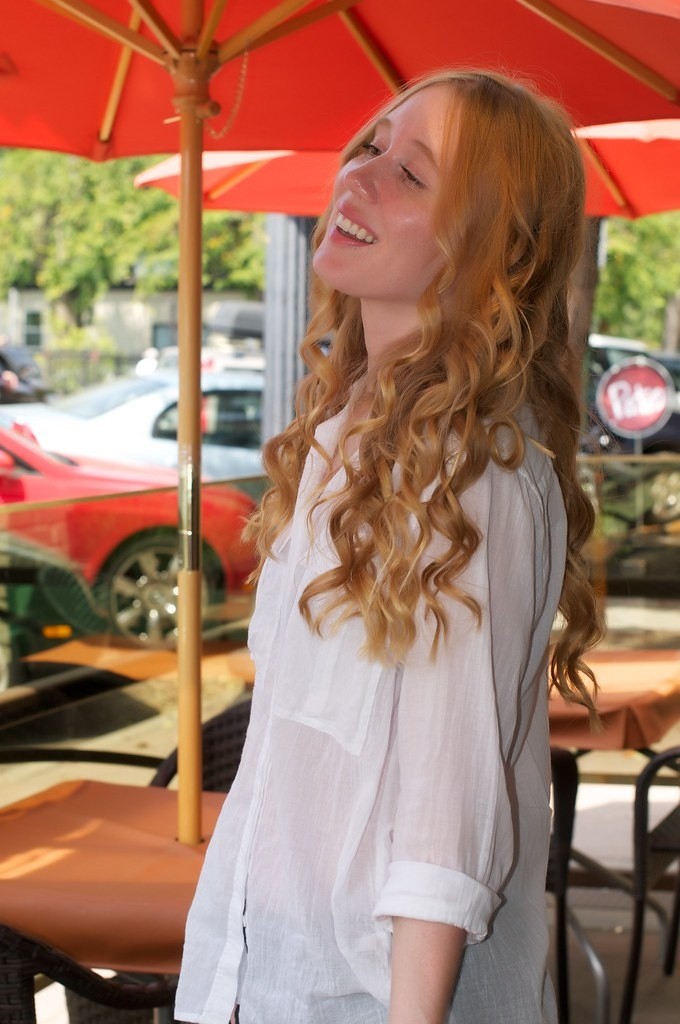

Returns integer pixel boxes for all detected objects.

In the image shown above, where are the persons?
[175,71,605,1023]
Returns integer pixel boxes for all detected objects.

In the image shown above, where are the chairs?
[0,924,178,1024]
[148,698,252,794]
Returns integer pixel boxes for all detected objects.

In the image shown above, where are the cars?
[0,336,56,403]
[0,364,175,444]
[0,419,277,690]
[38,373,273,482]
[587,336,680,451]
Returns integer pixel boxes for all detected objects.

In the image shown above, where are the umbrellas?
[2,0,679,846]
[136,121,680,223]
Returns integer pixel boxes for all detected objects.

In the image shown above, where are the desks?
[0,778,230,973]
[546,649,680,1024]
